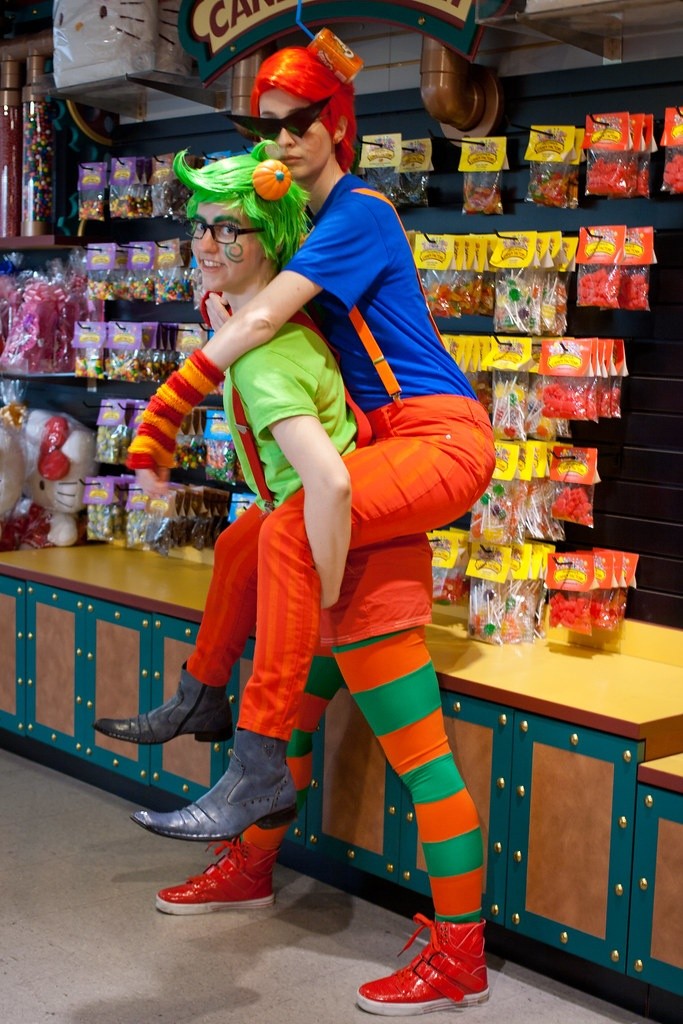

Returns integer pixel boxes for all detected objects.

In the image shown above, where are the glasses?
[222,94,333,140]
[181,218,265,245]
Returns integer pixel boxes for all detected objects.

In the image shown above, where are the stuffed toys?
[0,403,105,552]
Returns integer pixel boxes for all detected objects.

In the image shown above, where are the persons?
[158,139,490,1014]
[91,26,497,847]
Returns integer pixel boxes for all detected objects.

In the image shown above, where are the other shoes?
[130,728,298,843]
[92,661,235,745]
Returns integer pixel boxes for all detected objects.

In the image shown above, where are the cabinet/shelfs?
[1,540,683,1024]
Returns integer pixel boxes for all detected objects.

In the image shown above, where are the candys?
[87,427,237,554]
[88,275,195,304]
[75,354,180,383]
[79,193,153,219]
[21,101,55,221]
[374,155,683,646]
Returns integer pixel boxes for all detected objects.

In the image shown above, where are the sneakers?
[356,913,489,1017]
[155,839,276,915]
